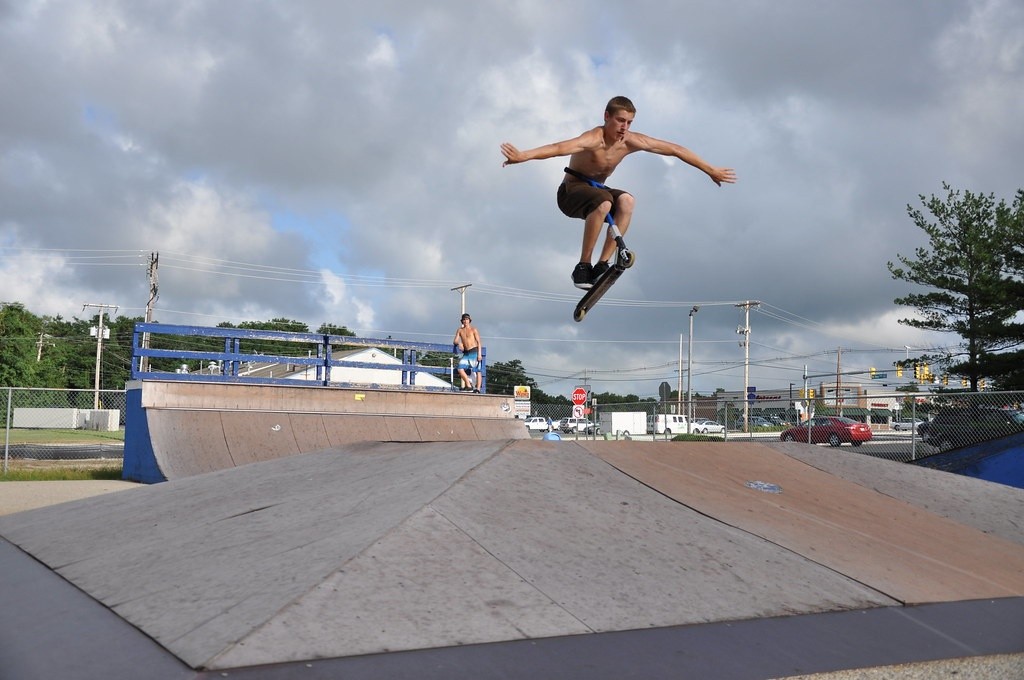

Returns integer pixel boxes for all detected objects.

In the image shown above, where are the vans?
[647,414,701,434]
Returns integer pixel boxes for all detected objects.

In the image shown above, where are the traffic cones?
[890,418,931,437]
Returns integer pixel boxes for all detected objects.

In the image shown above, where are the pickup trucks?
[525,417,554,433]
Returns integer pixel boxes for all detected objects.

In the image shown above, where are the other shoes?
[460,386,474,393]
[473,389,481,394]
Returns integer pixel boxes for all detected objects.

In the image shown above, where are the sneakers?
[592,260,614,281]
[571,262,596,291]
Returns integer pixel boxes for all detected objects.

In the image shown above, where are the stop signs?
[572,388,587,405]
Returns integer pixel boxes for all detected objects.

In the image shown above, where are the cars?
[780,416,873,447]
[554,419,562,431]
[692,418,725,434]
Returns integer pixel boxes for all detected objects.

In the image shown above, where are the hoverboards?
[564,167,635,323]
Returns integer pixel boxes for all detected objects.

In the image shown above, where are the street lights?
[790,383,795,422]
[687,306,699,434]
[827,388,850,417]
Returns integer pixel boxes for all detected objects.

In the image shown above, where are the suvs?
[560,417,600,434]
[922,407,1024,451]
[737,416,791,429]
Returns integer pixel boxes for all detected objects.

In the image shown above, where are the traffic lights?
[920,374,925,384]
[929,374,934,384]
[924,367,929,380]
[942,375,948,386]
[897,364,903,377]
[913,367,920,379]
[809,389,814,398]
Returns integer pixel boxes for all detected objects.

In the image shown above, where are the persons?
[547,417,553,432]
[452,313,482,392]
[501,96,736,292]
